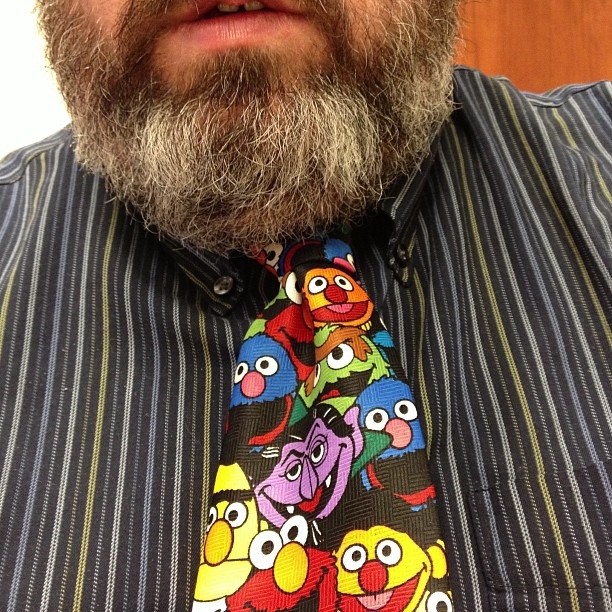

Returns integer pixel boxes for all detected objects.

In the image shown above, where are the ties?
[191,232,454,612]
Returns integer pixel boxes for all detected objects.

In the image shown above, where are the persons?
[1,1,611,610]
